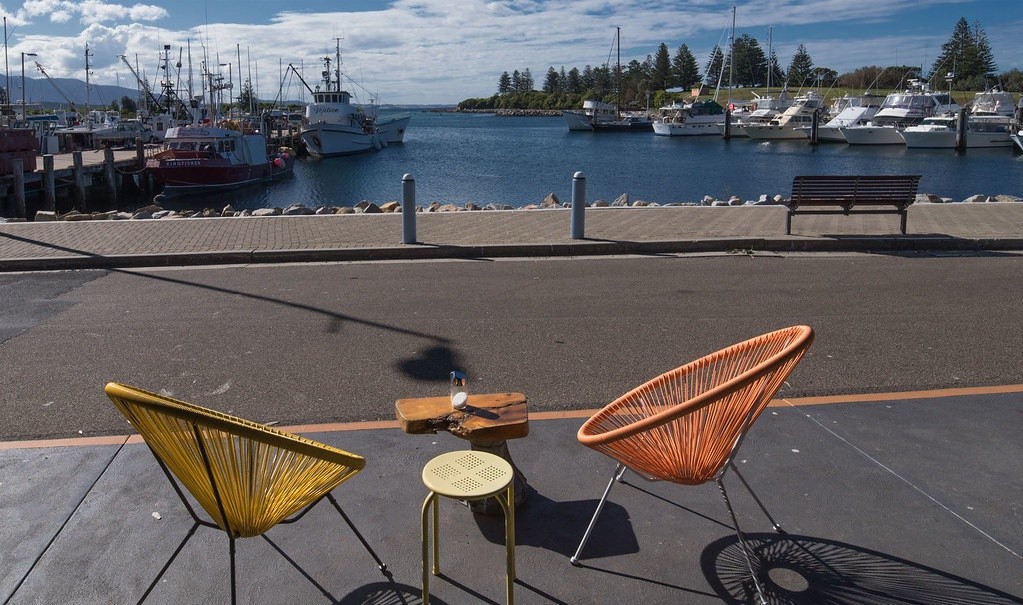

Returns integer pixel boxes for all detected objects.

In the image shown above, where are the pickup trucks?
[92,118,161,151]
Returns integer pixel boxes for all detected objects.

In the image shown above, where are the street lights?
[21,51,38,126]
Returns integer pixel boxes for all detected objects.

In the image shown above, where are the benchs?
[780,174,924,234]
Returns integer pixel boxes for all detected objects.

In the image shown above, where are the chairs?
[103,381,394,605]
[570,326,814,605]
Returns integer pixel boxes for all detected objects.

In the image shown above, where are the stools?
[421,450,515,605]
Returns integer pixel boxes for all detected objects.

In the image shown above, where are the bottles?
[450,369,469,411]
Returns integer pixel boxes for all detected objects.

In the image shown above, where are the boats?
[298,25,390,159]
[35,0,308,201]
[715,44,1023,154]
[364,95,413,143]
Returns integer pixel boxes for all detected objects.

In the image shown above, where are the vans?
[286,113,304,131]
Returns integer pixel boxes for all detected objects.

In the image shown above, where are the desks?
[395,391,530,515]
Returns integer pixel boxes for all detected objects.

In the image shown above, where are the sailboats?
[561,24,654,133]
[651,4,756,137]
